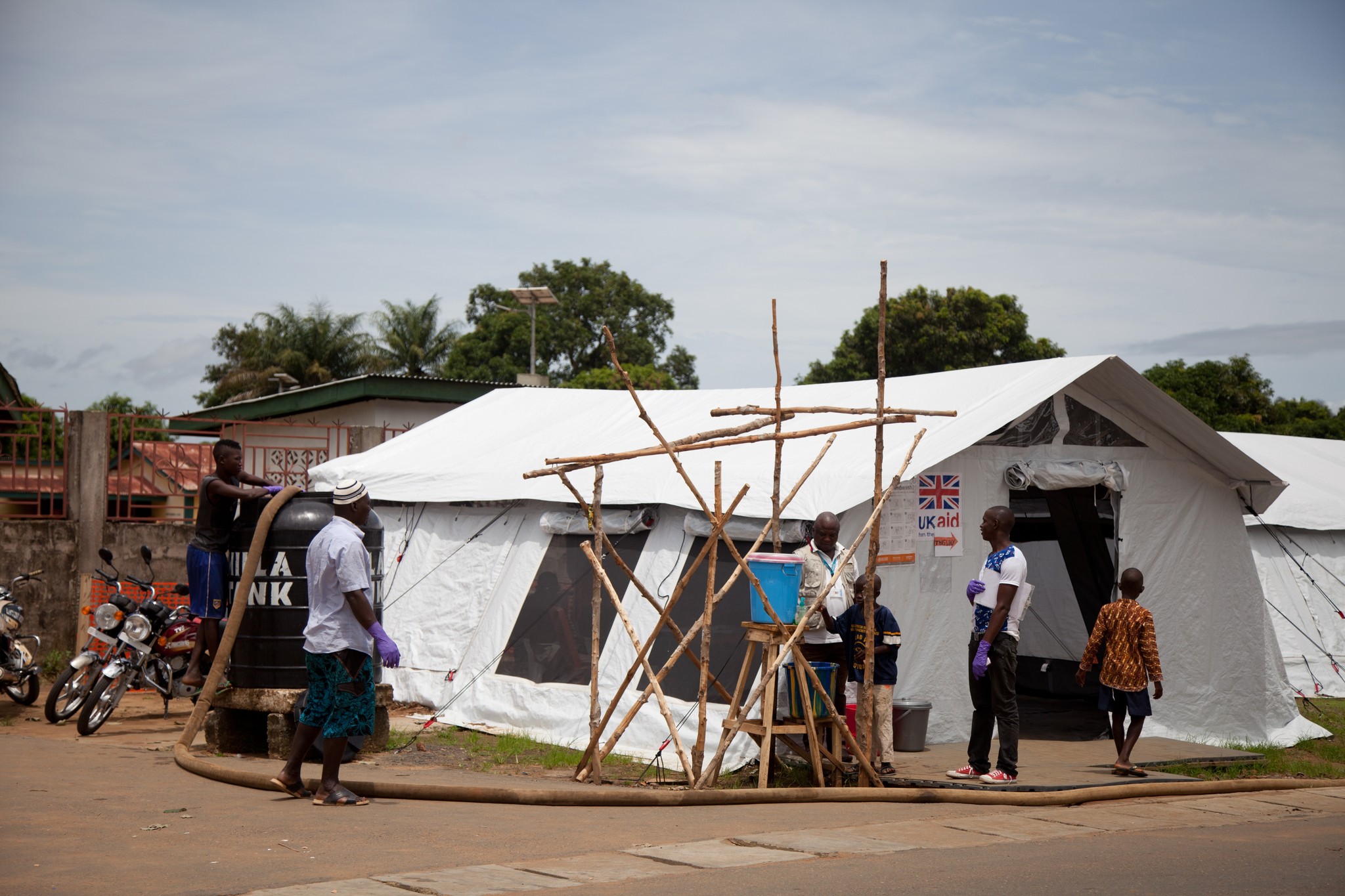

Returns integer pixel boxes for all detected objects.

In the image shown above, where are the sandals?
[844,760,879,775]
[880,764,896,776]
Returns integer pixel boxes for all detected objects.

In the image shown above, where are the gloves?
[966,580,986,600]
[367,621,401,669]
[973,640,991,680]
[263,485,306,497]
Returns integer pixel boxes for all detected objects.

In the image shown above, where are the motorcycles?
[44,545,228,736]
[0,570,48,705]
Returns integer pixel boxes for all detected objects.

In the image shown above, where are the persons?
[793,511,860,765]
[182,439,284,695]
[1074,567,1164,776]
[513,570,568,664]
[269,476,401,805]
[945,505,1028,785]
[814,572,902,776]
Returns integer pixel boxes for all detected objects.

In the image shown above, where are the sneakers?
[979,769,1017,785]
[946,765,984,779]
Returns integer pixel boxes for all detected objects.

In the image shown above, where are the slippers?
[215,680,233,695]
[1116,764,1148,777]
[1111,769,1129,776]
[185,686,203,698]
[269,778,314,798]
[312,787,369,806]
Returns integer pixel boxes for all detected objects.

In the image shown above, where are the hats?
[332,479,368,504]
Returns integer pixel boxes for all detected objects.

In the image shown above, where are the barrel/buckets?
[226,491,384,689]
[782,661,840,720]
[893,697,932,752]
[746,552,806,625]
[845,704,882,756]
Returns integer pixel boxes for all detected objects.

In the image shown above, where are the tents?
[305,351,1333,778]
[1217,428,1345,702]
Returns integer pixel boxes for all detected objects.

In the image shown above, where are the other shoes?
[841,744,853,763]
